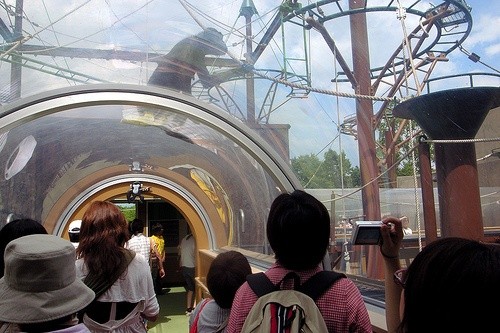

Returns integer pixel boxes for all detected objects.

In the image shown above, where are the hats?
[68,220,82,233]
[0,233,96,324]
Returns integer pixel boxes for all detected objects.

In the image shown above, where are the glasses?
[392,266,407,290]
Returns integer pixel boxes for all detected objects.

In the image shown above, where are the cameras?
[351,220,391,245]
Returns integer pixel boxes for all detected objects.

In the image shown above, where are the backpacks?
[240,270,347,333]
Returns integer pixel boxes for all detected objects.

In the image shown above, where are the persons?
[188,251,253,333]
[138,28,255,130]
[227,189,372,333]
[339,217,352,235]
[381,215,500,333]
[0,202,196,333]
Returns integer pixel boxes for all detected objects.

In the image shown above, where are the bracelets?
[381,251,400,258]
